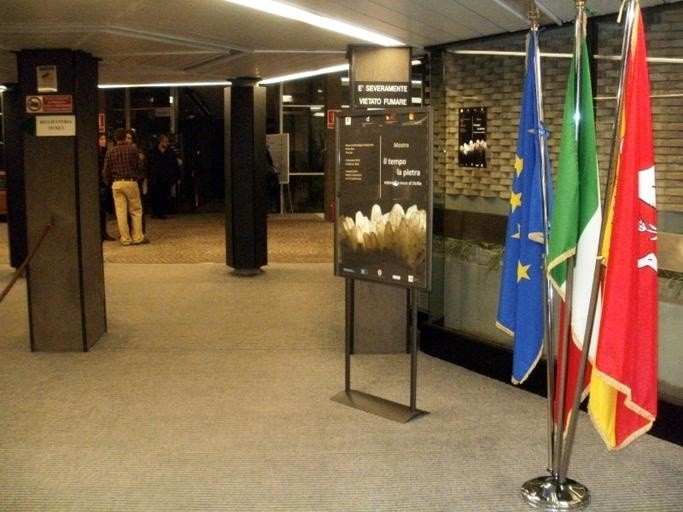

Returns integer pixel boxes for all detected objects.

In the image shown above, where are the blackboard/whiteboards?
[265,133,290,185]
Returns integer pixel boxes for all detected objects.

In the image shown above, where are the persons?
[99,128,177,246]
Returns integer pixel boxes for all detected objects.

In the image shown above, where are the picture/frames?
[327,110,340,130]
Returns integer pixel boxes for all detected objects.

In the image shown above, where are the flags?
[496,32,554,386]
[588,2,660,452]
[547,21,602,429]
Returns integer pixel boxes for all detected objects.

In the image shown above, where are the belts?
[112,178,138,182]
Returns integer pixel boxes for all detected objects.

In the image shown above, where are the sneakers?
[134,237,150,245]
